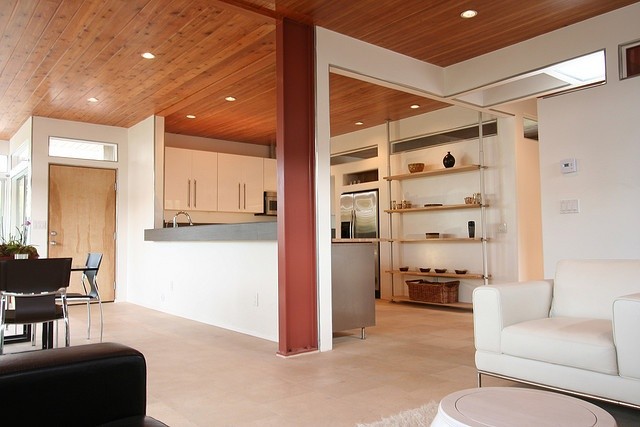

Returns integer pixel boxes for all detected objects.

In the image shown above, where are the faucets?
[173,211,193,227]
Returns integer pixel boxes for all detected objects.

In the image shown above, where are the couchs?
[0,340,169,426]
[470,258,636,410]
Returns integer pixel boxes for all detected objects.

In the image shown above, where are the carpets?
[355,399,438,427]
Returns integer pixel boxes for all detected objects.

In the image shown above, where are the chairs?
[1,254,72,354]
[55,250,105,348]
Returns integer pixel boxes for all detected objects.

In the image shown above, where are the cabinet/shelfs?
[383,162,493,312]
[218,150,264,215]
[163,146,217,213]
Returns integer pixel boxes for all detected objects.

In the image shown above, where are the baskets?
[405,279,460,303]
[408,163,424,173]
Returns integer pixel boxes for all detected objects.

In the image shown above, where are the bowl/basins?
[408,162,424,172]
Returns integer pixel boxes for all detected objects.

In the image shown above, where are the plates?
[455,269,467,274]
[434,269,447,273]
[400,267,409,271]
[424,204,442,207]
[420,268,431,272]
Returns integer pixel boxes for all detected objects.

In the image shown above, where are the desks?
[428,386,620,427]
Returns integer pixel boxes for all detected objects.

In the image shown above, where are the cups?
[468,221,475,239]
